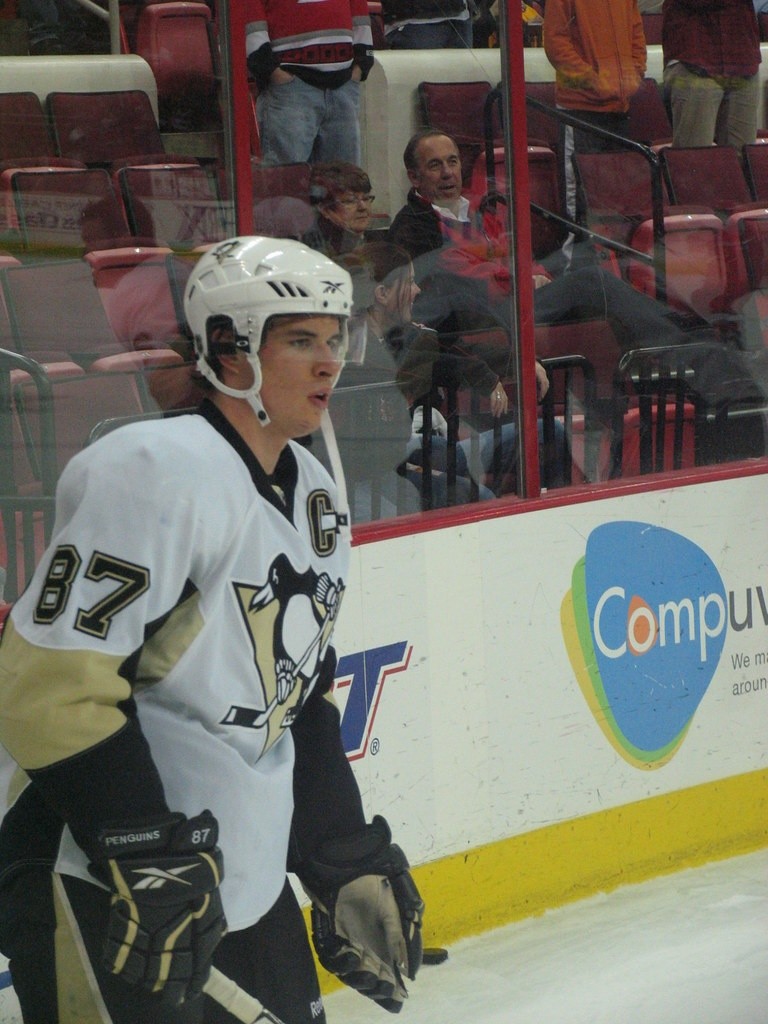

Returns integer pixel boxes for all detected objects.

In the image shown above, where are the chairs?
[0,0,768,619]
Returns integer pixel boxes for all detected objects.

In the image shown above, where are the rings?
[496,392,501,400]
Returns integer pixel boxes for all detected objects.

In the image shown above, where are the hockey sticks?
[201,964,287,1024]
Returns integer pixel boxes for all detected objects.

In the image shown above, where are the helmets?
[184,235,354,359]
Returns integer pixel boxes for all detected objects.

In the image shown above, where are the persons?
[290,159,549,523]
[0,234,423,1024]
[14,0,112,56]
[380,0,473,50]
[544,0,649,243]
[387,129,755,412]
[662,0,762,220]
[243,0,374,168]
[473,0,534,49]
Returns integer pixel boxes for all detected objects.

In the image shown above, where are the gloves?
[85,804,226,1024]
[289,815,425,1013]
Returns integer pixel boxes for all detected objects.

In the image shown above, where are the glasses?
[321,191,376,212]
